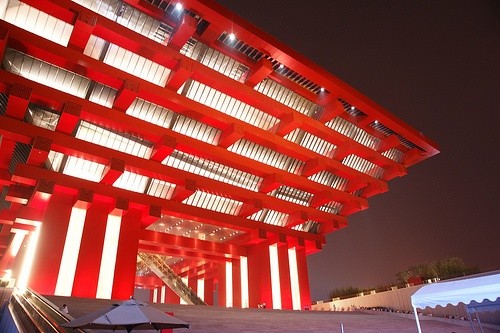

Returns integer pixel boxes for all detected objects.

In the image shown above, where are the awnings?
[411,273,500,333]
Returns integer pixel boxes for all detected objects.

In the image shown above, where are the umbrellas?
[60,297,191,329]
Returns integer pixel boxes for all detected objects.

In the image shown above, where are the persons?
[257,301,266,308]
[61,304,69,314]
[361,307,477,321]
